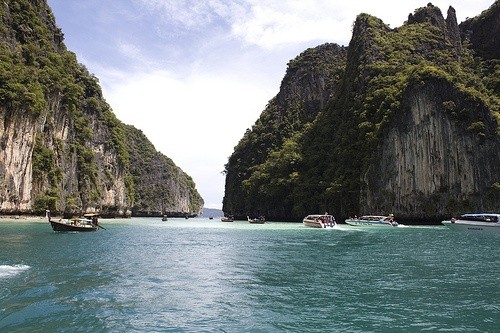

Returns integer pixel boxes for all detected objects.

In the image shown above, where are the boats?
[441,213,500,226]
[303,212,337,229]
[46,210,106,232]
[220,214,235,222]
[247,215,266,224]
[343,214,397,227]
